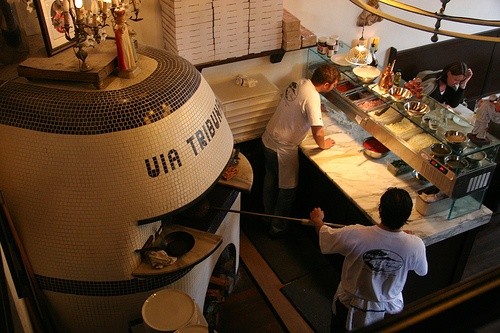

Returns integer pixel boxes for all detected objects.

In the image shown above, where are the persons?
[416,61,473,108]
[310,187,428,333]
[261,65,341,239]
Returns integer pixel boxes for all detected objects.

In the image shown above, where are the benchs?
[388,27,500,98]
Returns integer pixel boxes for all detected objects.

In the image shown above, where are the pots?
[135,230,195,258]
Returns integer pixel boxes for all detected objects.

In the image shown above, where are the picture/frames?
[33,0,86,57]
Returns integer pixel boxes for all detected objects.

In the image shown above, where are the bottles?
[394,72,401,84]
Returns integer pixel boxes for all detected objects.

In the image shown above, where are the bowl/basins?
[352,66,381,83]
[388,86,412,102]
[361,136,389,158]
[423,115,486,170]
[403,101,430,118]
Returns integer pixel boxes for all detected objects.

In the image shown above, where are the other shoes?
[268,226,288,239]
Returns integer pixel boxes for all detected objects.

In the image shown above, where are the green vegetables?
[390,159,408,168]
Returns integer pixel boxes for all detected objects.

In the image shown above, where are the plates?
[441,108,471,126]
[344,56,373,65]
[331,54,353,66]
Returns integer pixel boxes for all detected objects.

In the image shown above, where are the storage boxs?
[159,0,317,64]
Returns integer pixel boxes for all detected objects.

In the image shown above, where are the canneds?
[404,78,423,99]
[317,34,339,57]
